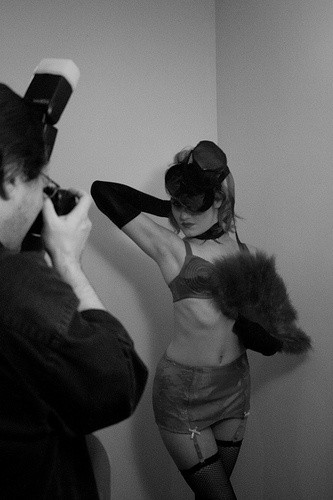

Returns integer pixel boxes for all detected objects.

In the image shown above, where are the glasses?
[39,171,60,199]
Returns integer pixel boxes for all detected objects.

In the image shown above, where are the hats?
[165,141,230,212]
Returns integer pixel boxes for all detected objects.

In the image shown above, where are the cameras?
[20,59,77,252]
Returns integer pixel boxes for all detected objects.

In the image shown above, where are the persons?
[0,83,148,500]
[90,140,313,500]
